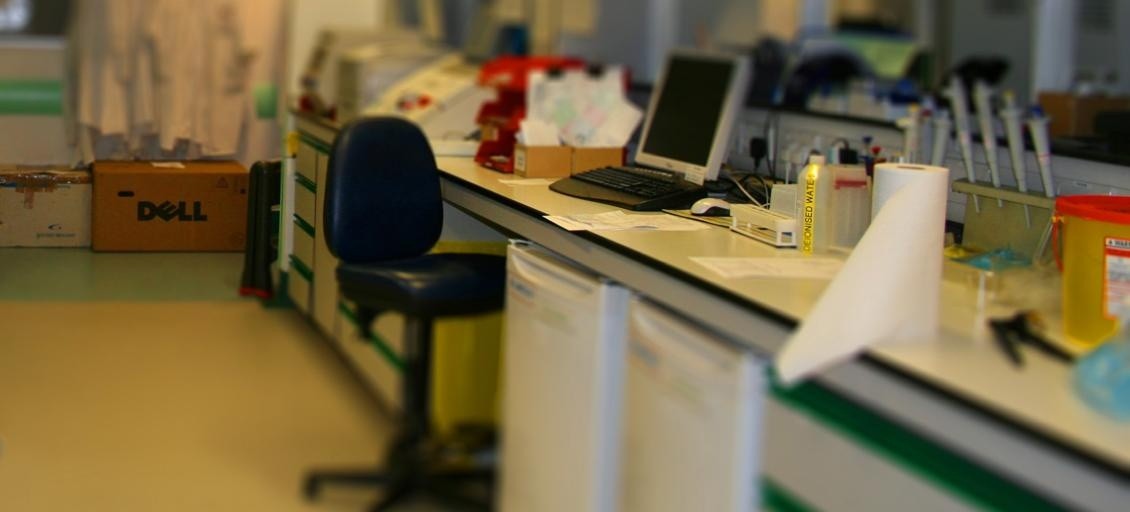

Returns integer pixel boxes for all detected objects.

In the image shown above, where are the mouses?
[690,196,730,219]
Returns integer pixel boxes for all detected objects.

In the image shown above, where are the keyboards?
[548,164,709,212]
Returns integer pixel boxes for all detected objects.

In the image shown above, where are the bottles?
[833,165,869,248]
[798,155,834,255]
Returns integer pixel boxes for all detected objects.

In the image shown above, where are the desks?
[283,103,1125,512]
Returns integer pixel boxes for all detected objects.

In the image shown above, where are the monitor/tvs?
[634,47,754,182]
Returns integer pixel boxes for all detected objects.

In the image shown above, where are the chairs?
[301,113,505,512]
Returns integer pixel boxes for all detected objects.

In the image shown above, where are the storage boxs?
[92,158,250,252]
[0,164,92,249]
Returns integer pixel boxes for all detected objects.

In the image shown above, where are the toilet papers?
[777,160,950,387]
